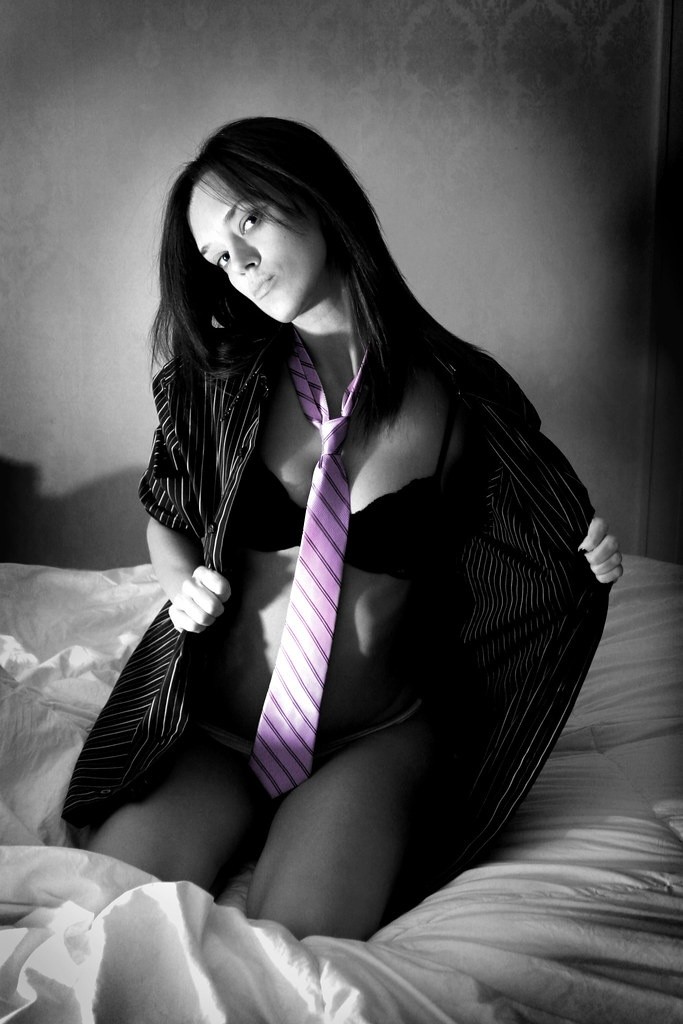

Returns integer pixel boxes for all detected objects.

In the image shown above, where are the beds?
[0,554,683,1024]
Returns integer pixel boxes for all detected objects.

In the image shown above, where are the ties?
[250,328,369,799]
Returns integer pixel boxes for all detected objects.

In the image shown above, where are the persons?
[59,114,624,939]
